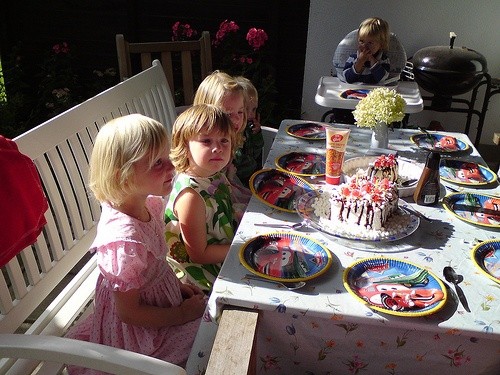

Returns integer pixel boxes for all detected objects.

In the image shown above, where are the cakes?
[314,154,403,234]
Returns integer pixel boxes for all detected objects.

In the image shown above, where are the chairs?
[315,29,424,129]
[115,31,213,113]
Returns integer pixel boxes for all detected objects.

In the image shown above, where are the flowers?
[172,18,271,84]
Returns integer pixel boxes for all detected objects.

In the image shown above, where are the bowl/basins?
[341,154,424,197]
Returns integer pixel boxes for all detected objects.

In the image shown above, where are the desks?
[187,120,500,375]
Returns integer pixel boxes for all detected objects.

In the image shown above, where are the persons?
[162,103,240,297]
[62,112,209,375]
[343,17,391,124]
[194,70,252,225]
[233,76,265,187]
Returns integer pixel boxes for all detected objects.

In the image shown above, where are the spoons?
[245,274,306,291]
[400,177,418,187]
[254,223,304,230]
[443,267,470,313]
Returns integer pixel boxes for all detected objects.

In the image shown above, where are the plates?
[239,232,333,283]
[439,158,498,186]
[275,148,326,177]
[343,258,447,317]
[409,132,471,155]
[442,190,500,229]
[295,187,421,244]
[472,238,500,285]
[340,88,372,102]
[286,121,335,141]
[249,168,318,214]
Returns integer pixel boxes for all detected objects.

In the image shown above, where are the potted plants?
[351,86,408,148]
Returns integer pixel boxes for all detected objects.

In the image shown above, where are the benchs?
[0,58,206,375]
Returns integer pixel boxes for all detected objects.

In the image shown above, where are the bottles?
[413,150,441,206]
